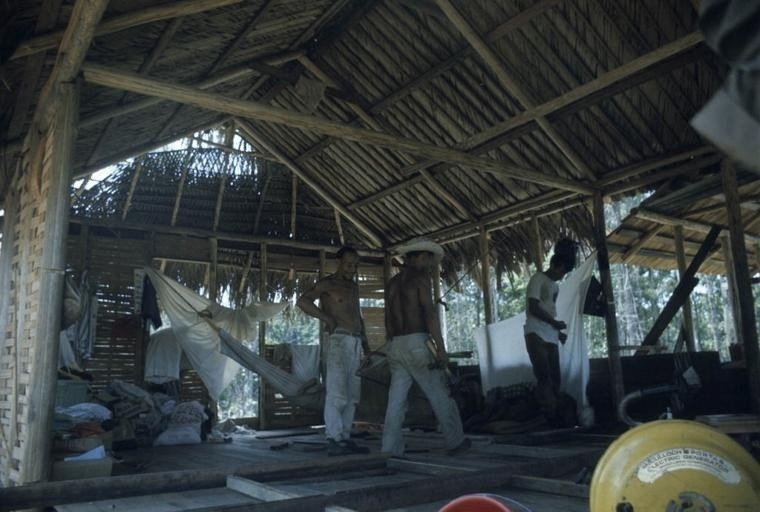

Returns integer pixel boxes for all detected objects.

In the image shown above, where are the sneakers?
[326,436,358,455]
[447,436,471,457]
[381,440,408,458]
[347,437,371,454]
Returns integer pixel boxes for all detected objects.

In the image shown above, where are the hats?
[397,235,446,264]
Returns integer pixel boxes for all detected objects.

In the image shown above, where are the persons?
[379,236,474,469]
[523,254,574,430]
[294,244,375,459]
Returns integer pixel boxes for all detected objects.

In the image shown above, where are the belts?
[336,329,361,337]
